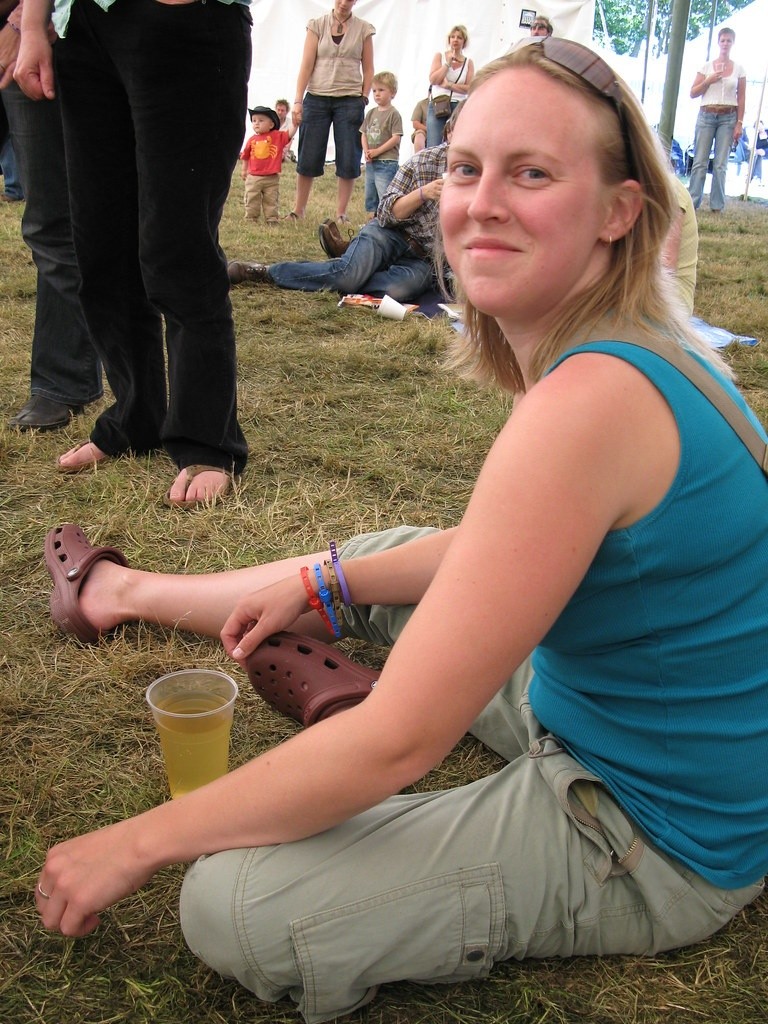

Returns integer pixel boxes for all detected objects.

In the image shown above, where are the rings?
[37,882,52,900]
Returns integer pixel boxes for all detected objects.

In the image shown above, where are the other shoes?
[228,260,271,284]
[12,394,82,430]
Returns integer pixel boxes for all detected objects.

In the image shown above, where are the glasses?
[501,36,624,121]
[531,23,549,30]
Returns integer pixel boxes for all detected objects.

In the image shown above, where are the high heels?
[318,218,349,260]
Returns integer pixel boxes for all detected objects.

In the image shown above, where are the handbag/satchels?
[431,94,451,119]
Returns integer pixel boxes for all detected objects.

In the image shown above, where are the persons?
[227,119,452,301]
[241,101,302,226]
[410,26,475,153]
[34,35,768,1024]
[14,0,253,508]
[745,121,768,187]
[282,0,375,226]
[359,71,404,219]
[688,28,747,212]
[0,0,104,431]
[531,16,553,35]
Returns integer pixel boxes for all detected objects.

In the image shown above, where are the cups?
[376,294,407,321]
[145,669,239,800]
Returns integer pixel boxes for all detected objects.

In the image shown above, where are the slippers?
[55,443,109,473]
[246,631,381,730]
[163,465,235,509]
[44,523,130,643]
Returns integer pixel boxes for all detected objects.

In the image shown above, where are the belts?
[700,105,737,113]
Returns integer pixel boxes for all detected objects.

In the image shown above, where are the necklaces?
[333,15,352,33]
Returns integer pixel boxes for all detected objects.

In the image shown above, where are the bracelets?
[293,98,304,104]
[703,81,709,88]
[299,540,354,637]
[445,61,451,67]
[738,119,744,124]
[419,184,426,202]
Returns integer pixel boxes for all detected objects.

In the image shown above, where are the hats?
[246,105,280,131]
